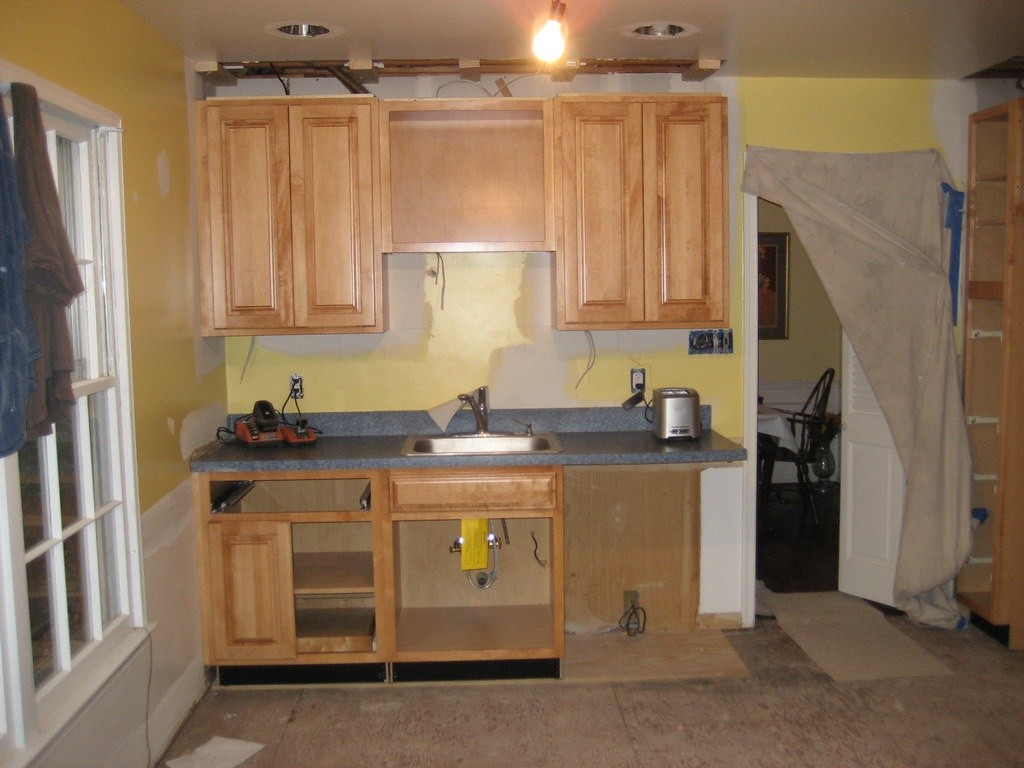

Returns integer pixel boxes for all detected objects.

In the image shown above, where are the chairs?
[760,367,836,529]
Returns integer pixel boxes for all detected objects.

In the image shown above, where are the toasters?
[652,386,702,445]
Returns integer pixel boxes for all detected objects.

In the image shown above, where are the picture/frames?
[758,232,790,340]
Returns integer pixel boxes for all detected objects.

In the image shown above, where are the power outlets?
[288,372,304,399]
[631,367,646,393]
[623,591,640,625]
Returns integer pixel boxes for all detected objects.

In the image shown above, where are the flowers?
[805,411,842,439]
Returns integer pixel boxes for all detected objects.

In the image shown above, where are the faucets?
[457,385,489,434]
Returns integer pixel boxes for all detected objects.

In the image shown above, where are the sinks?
[400,430,564,456]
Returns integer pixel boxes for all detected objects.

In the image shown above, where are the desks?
[754,404,798,455]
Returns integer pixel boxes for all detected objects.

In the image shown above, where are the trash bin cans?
[765,481,840,592]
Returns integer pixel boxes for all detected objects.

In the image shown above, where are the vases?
[812,433,836,491]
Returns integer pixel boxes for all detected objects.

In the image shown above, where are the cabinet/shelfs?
[196,464,566,684]
[951,96,1024,625]
[552,92,730,331]
[193,98,391,337]
